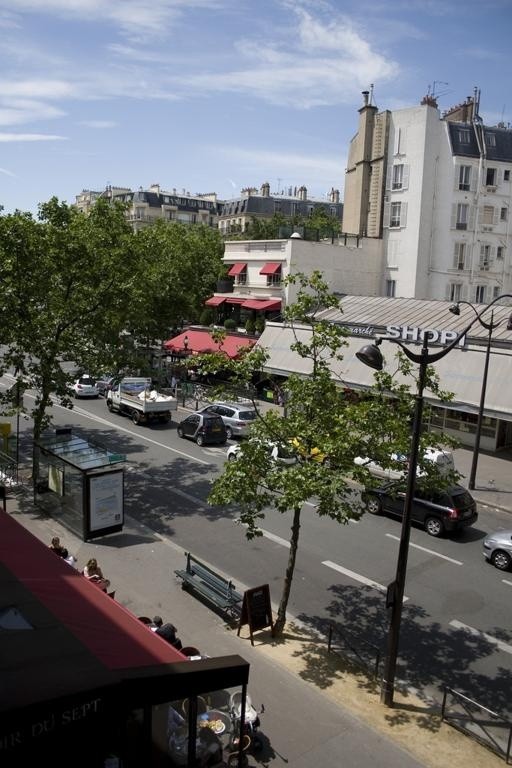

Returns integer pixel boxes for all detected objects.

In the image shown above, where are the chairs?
[182,695,208,720]
[213,734,252,768]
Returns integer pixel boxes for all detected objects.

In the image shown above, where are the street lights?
[11,373,27,489]
[356,294,512,709]
[448,300,512,490]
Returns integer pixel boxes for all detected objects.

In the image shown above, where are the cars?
[64,372,121,399]
[177,411,229,447]
[481,528,512,571]
[225,431,346,474]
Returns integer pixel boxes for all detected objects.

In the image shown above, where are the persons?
[153,616,177,643]
[198,727,223,768]
[84,558,103,580]
[48,536,68,559]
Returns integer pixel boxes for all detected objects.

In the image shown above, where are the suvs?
[196,402,260,441]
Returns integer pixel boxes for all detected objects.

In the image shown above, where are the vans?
[361,473,479,539]
[352,444,456,480]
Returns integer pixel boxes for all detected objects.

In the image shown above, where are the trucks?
[103,375,179,424]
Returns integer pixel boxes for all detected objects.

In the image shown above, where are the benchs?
[174,550,243,619]
